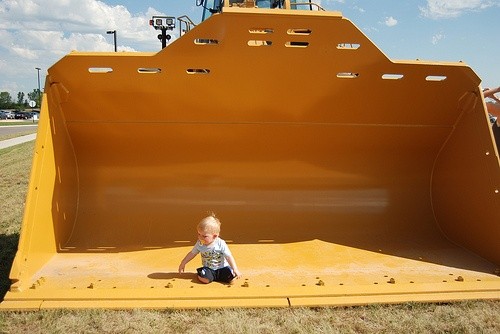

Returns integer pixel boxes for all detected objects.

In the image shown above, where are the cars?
[0,109,41,120]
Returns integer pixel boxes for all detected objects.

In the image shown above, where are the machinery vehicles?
[0,0,500,313]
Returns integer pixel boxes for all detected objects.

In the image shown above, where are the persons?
[179,217,241,285]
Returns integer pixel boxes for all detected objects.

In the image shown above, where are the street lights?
[35,67,41,110]
[106,29,117,52]
